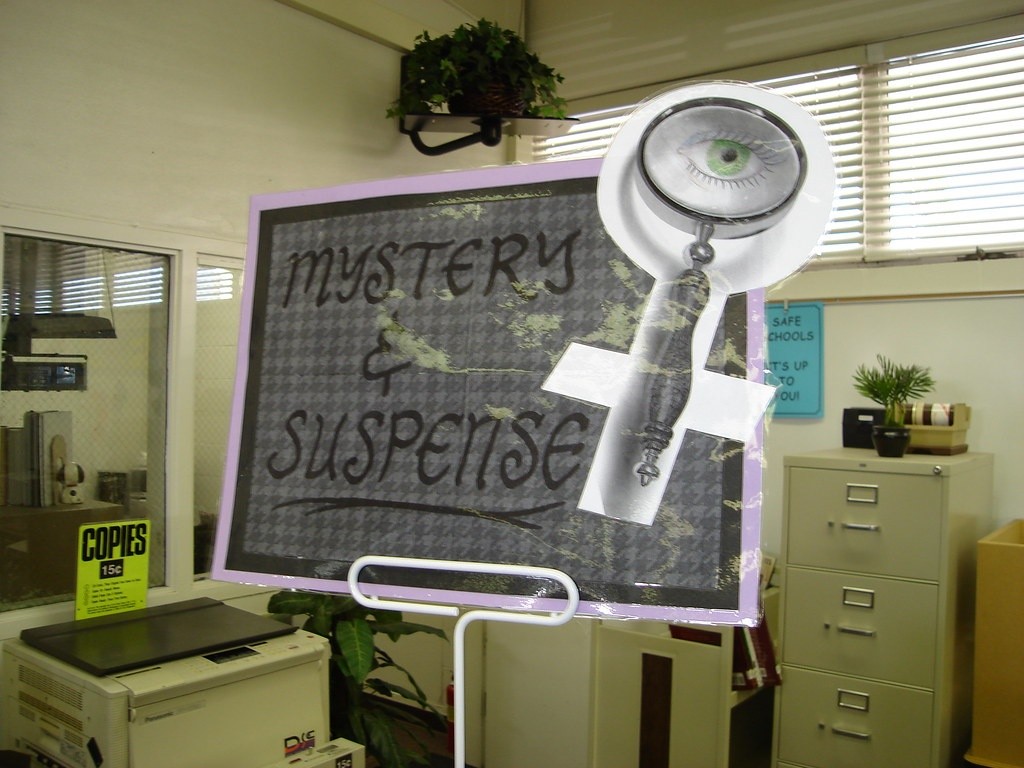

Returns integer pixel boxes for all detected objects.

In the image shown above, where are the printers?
[0,595,366,768]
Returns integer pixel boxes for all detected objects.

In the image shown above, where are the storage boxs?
[0,497,126,603]
[965,516,1024,768]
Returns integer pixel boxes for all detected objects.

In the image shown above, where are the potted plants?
[852,353,934,457]
[383,16,569,121]
[267,590,450,768]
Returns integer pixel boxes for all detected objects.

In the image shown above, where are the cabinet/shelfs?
[771,453,999,768]
[586,574,781,767]
